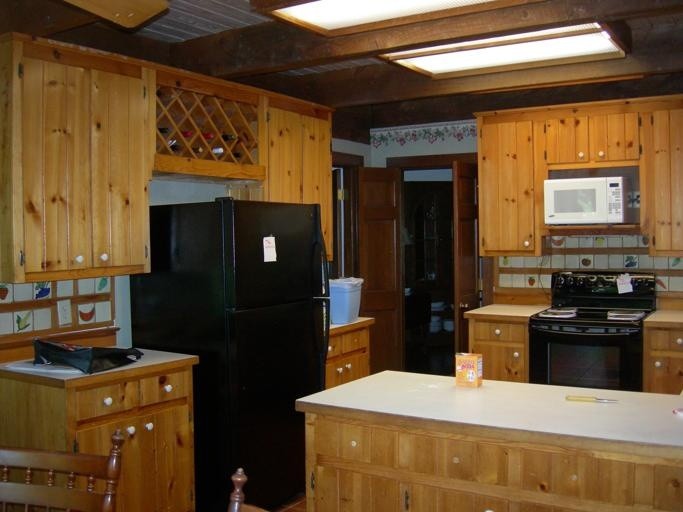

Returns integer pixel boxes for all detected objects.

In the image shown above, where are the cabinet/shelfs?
[292,368,681,512]
[152,62,262,185]
[226,87,334,263]
[544,98,647,233]
[647,92,682,257]
[0,345,201,511]
[324,316,377,391]
[461,313,531,389]
[640,320,682,396]
[471,106,543,256]
[0,31,152,286]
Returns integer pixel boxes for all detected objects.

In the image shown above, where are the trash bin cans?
[329,277,364,324]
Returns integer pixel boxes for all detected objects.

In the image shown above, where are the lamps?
[374,17,634,81]
[245,0,549,43]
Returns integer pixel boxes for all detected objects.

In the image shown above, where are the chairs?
[0,426,269,512]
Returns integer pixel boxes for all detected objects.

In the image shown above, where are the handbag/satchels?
[33,339,143,374]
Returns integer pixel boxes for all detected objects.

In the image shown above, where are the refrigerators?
[130,197,331,512]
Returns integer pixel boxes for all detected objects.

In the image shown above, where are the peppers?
[79,310,93,321]
[97,277,108,291]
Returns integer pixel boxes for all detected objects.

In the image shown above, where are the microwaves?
[543,177,630,224]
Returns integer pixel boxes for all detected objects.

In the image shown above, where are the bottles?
[155,125,242,160]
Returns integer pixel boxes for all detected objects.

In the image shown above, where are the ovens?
[528,323,642,392]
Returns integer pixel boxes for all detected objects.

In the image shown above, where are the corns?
[16,311,31,332]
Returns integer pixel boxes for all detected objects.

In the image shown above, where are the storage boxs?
[325,274,365,325]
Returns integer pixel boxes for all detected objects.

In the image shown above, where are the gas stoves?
[529,307,650,321]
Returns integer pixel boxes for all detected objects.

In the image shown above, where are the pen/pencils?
[566,395,619,403]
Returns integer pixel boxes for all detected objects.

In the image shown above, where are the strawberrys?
[0,287,8,300]
